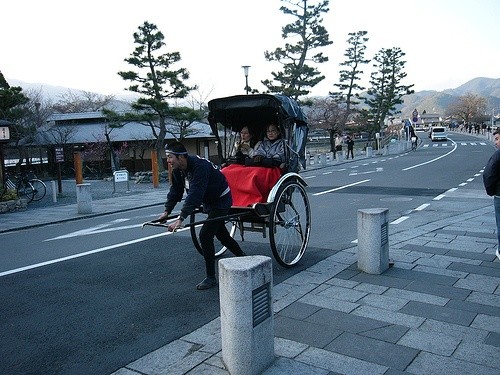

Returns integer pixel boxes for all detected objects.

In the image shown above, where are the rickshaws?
[139,92,313,269]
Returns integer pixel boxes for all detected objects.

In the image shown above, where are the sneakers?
[196,277,218,290]
[496,248,500,260]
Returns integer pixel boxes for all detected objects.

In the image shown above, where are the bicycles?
[0,170,47,205]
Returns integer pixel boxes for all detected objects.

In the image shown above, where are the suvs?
[428,125,448,141]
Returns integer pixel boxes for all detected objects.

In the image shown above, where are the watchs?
[177,215,184,221]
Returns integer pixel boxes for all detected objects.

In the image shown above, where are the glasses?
[266,129,281,133]
[239,131,253,135]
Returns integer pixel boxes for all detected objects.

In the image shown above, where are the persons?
[335,133,344,151]
[158,142,248,290]
[410,128,418,148]
[252,124,286,168]
[346,136,354,160]
[227,126,262,166]
[449,122,491,134]
[483,127,500,261]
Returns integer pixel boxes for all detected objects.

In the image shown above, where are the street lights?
[241,66,253,95]
